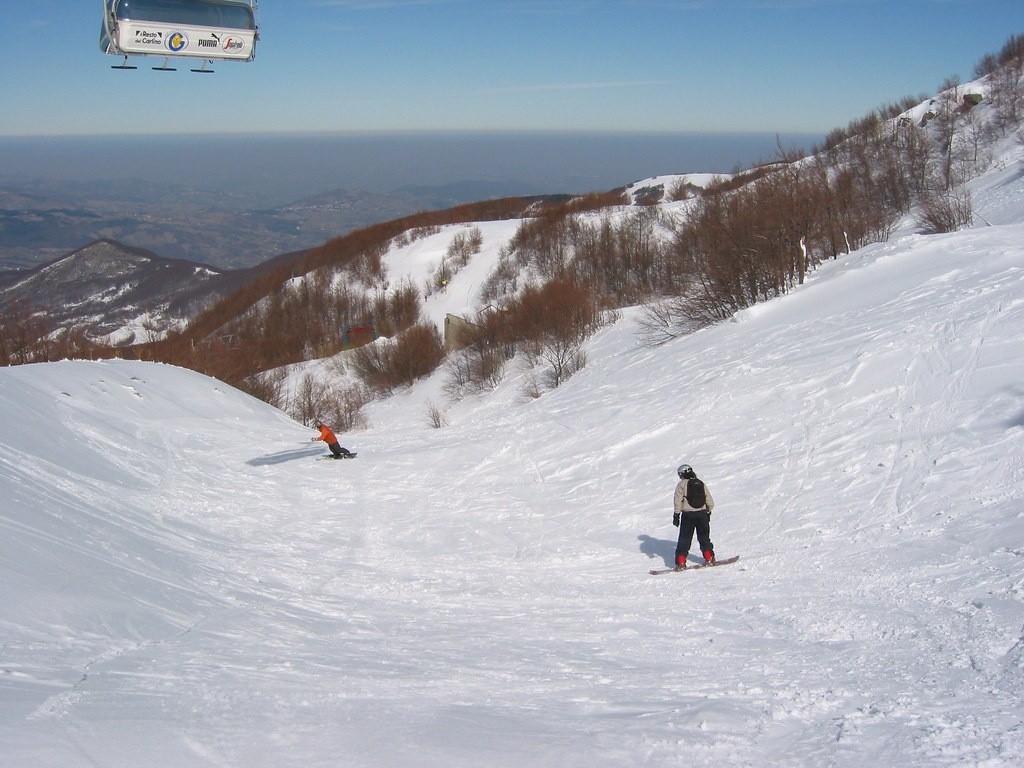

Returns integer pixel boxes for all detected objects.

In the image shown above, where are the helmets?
[678,464,692,475]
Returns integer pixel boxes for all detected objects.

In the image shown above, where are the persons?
[673,465,715,572]
[312,421,349,458]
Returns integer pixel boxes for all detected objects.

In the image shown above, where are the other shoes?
[334,454,340,457]
[344,453,350,458]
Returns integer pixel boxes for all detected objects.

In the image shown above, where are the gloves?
[673,512,680,527]
[311,438,317,441]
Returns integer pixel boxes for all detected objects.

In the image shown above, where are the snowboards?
[649,555,739,576]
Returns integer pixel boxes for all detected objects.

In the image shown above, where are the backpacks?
[684,478,706,508]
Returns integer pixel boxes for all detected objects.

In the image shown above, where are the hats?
[315,421,321,427]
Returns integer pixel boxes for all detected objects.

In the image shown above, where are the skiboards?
[315,452,357,460]
